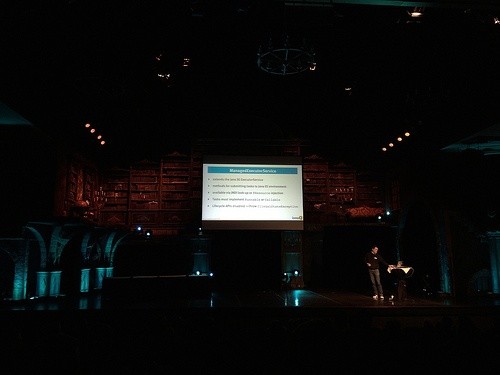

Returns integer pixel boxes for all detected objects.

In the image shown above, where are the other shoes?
[380,295,384,299]
[373,295,378,298]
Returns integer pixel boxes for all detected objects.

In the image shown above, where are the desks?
[102,273,212,303]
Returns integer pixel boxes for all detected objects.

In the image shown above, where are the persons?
[366,246,390,300]
